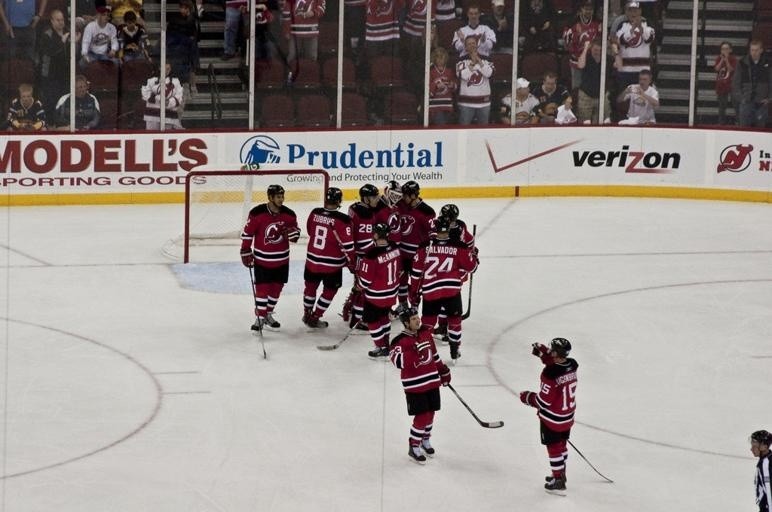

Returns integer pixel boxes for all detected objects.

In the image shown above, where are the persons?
[343,180,480,360]
[519,338,578,491]
[3,1,199,130]
[213,2,666,127]
[241,185,301,331]
[302,188,357,329]
[390,308,451,462]
[714,41,772,131]
[750,430,772,512]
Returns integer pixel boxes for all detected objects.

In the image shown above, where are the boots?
[450,345,461,359]
[433,324,447,334]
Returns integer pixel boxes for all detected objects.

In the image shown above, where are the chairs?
[1,53,159,130]
[257,17,423,126]
[749,0,772,53]
[488,47,632,123]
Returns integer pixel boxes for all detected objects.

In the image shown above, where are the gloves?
[531,342,551,357]
[440,364,451,386]
[240,248,255,268]
[408,291,420,306]
[520,390,539,409]
[472,247,479,265]
[415,339,431,355]
[286,226,301,243]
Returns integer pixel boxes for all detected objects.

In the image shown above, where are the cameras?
[628,84,640,93]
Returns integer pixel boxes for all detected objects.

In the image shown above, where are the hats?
[624,0,644,9]
[491,0,505,7]
[96,6,110,14]
[516,78,530,89]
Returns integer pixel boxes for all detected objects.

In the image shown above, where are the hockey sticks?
[249,267,267,361]
[317,320,363,350]
[567,440,614,484]
[447,382,504,428]
[460,224,474,320]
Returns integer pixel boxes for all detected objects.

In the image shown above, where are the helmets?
[551,338,571,356]
[326,188,342,199]
[751,429,772,445]
[268,185,284,194]
[402,181,419,193]
[399,308,418,322]
[359,184,378,196]
[436,216,450,228]
[441,204,458,219]
[385,181,403,204]
[373,223,391,234]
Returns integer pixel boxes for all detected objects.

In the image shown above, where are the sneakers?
[251,318,263,330]
[369,348,389,356]
[544,479,565,490]
[264,313,280,327]
[545,476,566,482]
[392,302,408,315]
[421,439,434,454]
[350,318,369,330]
[408,444,426,461]
[302,315,328,328]
[221,54,235,60]
